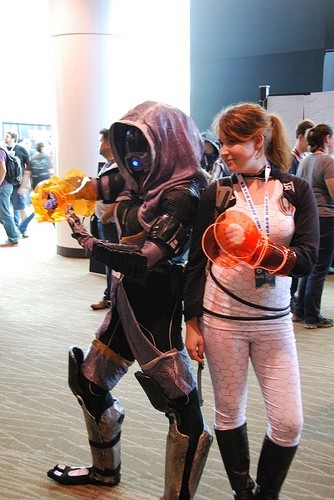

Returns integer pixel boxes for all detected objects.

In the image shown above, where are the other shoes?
[90,299,110,309]
[0,241,18,247]
[22,232,29,238]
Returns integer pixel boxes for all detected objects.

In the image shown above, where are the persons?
[291,123,334,329]
[288,120,315,176]
[32,100,214,500]
[184,103,320,500]
[90,128,120,310]
[5,132,32,222]
[201,129,221,172]
[19,143,56,238]
[0,146,19,248]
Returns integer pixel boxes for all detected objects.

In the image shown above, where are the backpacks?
[0,148,22,185]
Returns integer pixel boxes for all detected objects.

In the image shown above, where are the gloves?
[65,204,112,252]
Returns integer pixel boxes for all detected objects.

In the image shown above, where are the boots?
[159,412,213,500]
[254,433,299,500]
[215,423,255,500]
[47,348,125,486]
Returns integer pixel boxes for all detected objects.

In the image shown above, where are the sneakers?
[304,318,334,329]
[292,313,301,321]
